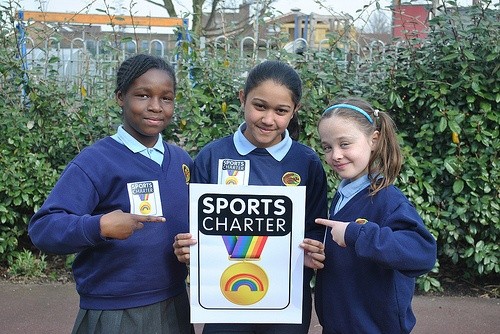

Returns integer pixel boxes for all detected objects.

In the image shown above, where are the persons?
[28,55,199,334]
[314,96,438,334]
[172,61,330,334]
